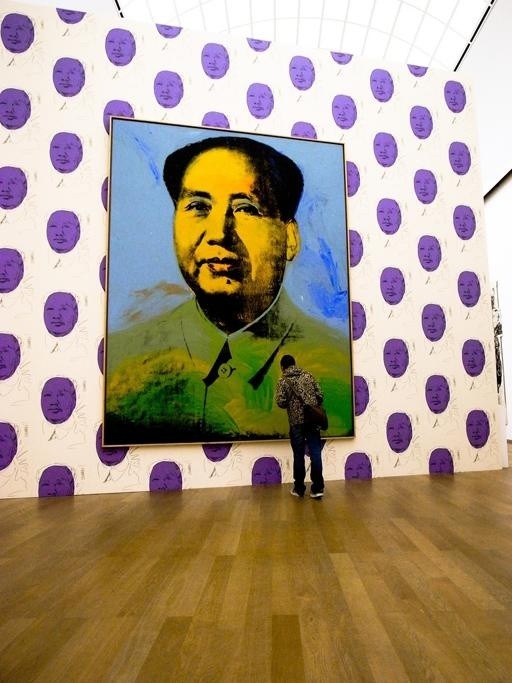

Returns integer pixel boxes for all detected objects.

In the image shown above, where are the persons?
[273,354,331,498]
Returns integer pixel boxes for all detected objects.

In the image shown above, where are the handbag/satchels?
[303,404,328,430]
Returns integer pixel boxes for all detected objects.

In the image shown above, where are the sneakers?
[291,490,304,497]
[309,491,324,498]
